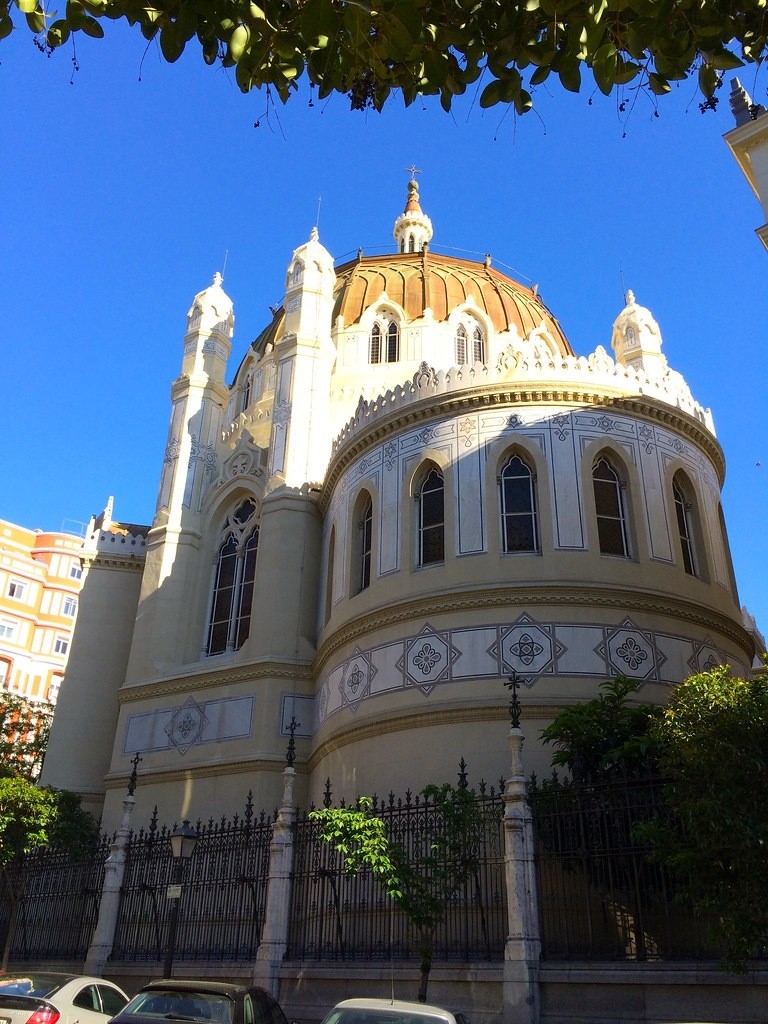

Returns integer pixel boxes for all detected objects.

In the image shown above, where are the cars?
[0,973,131,1024]
[321,997,471,1024]
[108,978,299,1024]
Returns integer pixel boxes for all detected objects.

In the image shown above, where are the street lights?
[162,820,199,981]
